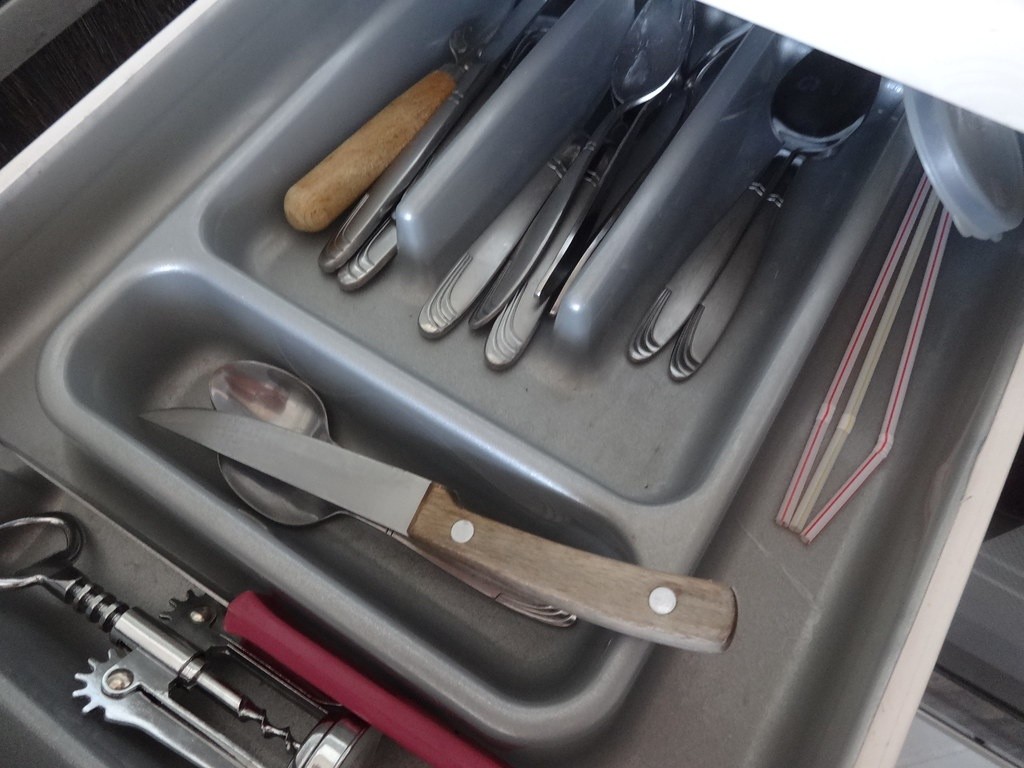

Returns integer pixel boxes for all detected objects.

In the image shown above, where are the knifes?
[209,386,576,629]
[318,0,550,274]
[138,408,735,652]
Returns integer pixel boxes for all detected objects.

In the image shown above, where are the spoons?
[418,0,885,383]
[208,356,576,626]
[213,451,576,629]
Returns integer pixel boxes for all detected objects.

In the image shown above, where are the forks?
[278,15,547,293]
